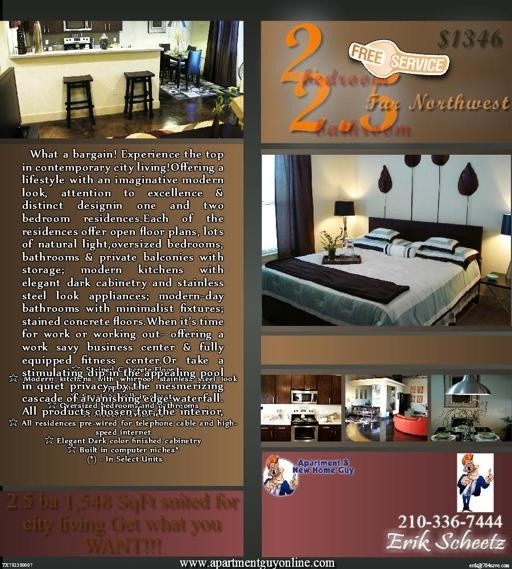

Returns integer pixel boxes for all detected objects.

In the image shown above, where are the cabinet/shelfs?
[261,374,343,442]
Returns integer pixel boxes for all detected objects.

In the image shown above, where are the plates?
[431,427,500,442]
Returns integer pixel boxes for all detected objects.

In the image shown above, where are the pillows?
[353,226,481,272]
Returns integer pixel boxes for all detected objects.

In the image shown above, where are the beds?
[262,218,485,329]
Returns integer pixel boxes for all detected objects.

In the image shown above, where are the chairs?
[158,44,202,90]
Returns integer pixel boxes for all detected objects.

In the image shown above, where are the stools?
[123,70,155,121]
[63,74,96,128]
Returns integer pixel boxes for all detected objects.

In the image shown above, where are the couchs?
[393,412,428,437]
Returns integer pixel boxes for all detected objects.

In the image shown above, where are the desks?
[430,426,498,444]
[477,270,512,328]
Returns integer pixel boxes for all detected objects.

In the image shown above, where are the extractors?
[291,389,318,405]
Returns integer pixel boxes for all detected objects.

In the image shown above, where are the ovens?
[291,425,318,443]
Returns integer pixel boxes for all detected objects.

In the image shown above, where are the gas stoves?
[292,416,317,424]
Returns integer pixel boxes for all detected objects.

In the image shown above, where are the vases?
[327,248,336,260]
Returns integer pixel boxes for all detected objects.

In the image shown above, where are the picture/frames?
[443,375,480,409]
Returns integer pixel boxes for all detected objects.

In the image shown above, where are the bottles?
[345,242,354,261]
[100,37,109,50]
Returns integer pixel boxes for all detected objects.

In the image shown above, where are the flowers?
[320,227,346,249]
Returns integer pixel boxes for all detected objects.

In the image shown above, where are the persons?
[458,454,495,512]
[263,454,297,496]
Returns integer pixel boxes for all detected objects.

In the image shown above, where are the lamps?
[444,375,492,398]
[333,200,355,245]
[499,211,511,282]
